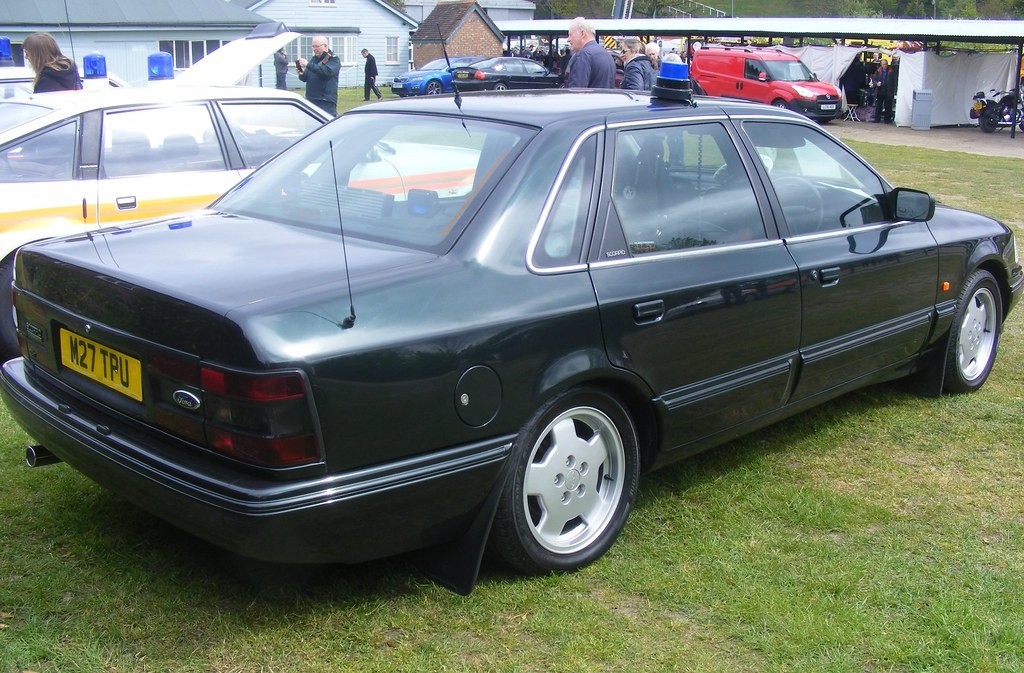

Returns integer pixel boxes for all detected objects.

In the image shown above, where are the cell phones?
[295,59,303,73]
[280,50,288,54]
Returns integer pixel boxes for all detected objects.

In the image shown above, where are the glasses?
[622,49,632,54]
[312,44,326,49]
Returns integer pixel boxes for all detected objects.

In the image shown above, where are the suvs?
[690,46,843,124]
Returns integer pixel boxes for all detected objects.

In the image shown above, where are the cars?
[0,62,1024,595]
[0,22,481,362]
[391,55,487,97]
[561,50,625,90]
[452,57,557,93]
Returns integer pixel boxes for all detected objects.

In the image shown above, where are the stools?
[844,103,860,122]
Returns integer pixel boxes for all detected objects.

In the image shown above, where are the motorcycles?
[970,88,1024,133]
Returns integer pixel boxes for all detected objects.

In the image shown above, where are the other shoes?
[885,120,889,124]
[378,96,383,101]
[363,98,370,101]
[872,120,878,123]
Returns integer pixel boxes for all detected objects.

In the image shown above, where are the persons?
[619,39,654,91]
[670,48,675,53]
[507,43,572,72]
[873,53,880,62]
[872,59,896,124]
[22,32,83,94]
[680,51,686,63]
[567,17,616,89]
[644,42,660,71]
[295,36,341,117]
[361,49,383,102]
[663,53,684,167]
[274,47,289,90]
